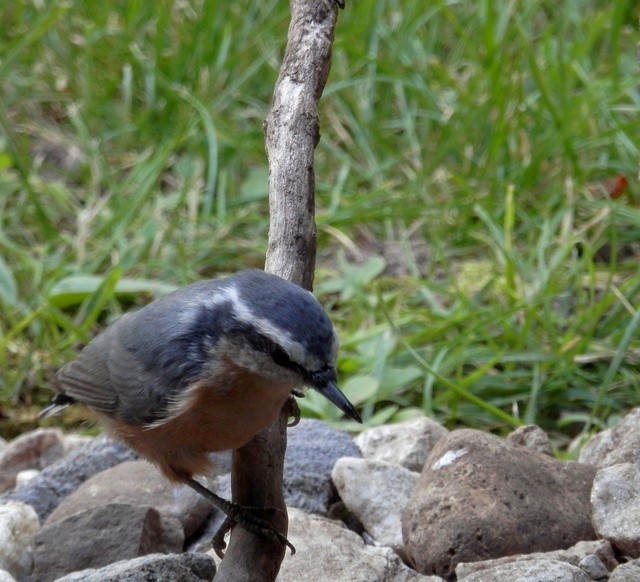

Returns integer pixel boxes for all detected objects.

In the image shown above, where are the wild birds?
[35,269,362,557]
